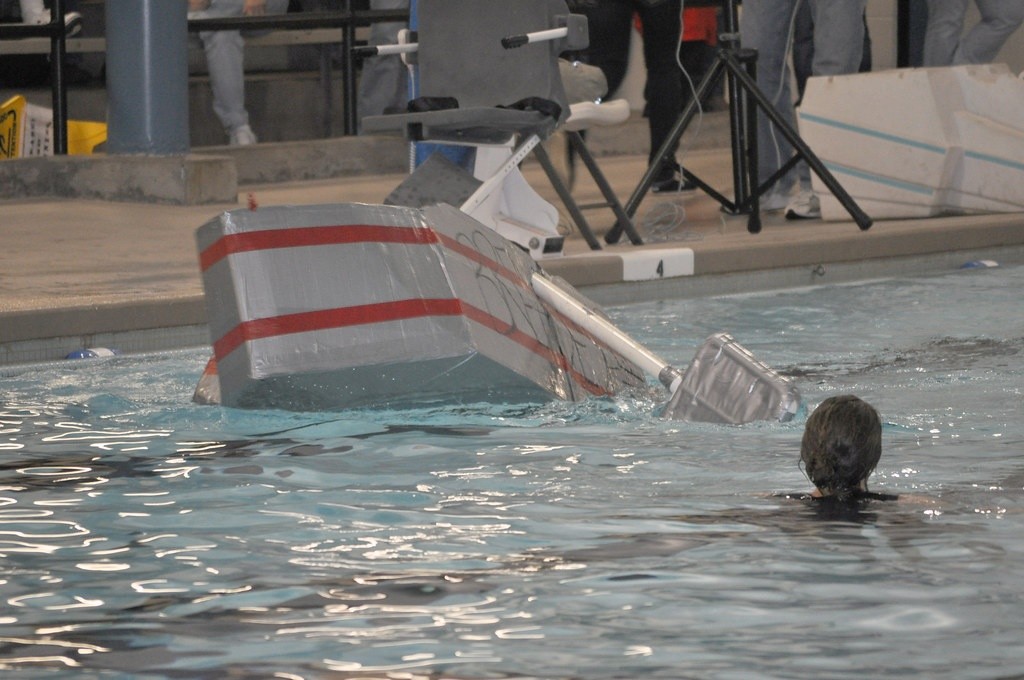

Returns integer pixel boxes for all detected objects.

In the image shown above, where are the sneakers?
[784,188,821,218]
[720,194,789,215]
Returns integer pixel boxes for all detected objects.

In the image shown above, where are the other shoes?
[653,179,696,195]
[229,125,256,147]
[28,9,82,38]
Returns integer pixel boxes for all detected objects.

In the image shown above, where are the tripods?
[604,0,876,245]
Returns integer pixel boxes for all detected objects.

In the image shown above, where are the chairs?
[351,0,642,250]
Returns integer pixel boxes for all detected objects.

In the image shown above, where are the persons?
[924,0,1024,66]
[782,396,908,502]
[722,0,871,218]
[19,0,82,38]
[189,0,287,146]
[566,0,716,193]
